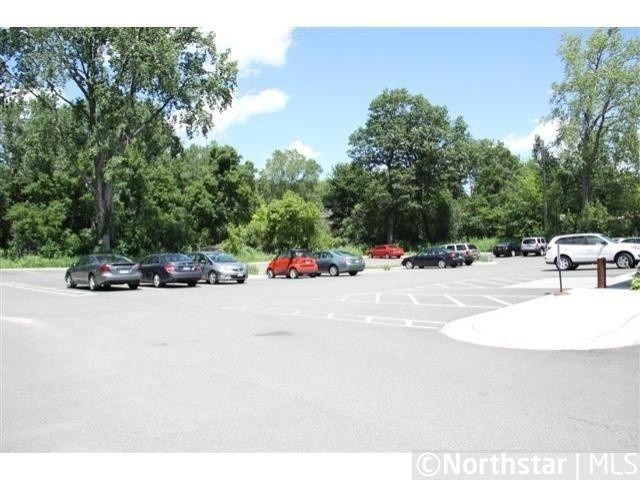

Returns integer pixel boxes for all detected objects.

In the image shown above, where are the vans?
[439,244,479,265]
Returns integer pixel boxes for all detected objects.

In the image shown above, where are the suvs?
[494,233,640,269]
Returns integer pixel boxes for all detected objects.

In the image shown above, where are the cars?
[367,244,405,259]
[65,253,144,294]
[401,249,467,269]
[189,251,248,287]
[267,247,318,278]
[135,253,202,290]
[313,250,365,276]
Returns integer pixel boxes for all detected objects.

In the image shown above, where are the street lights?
[534,140,550,233]
